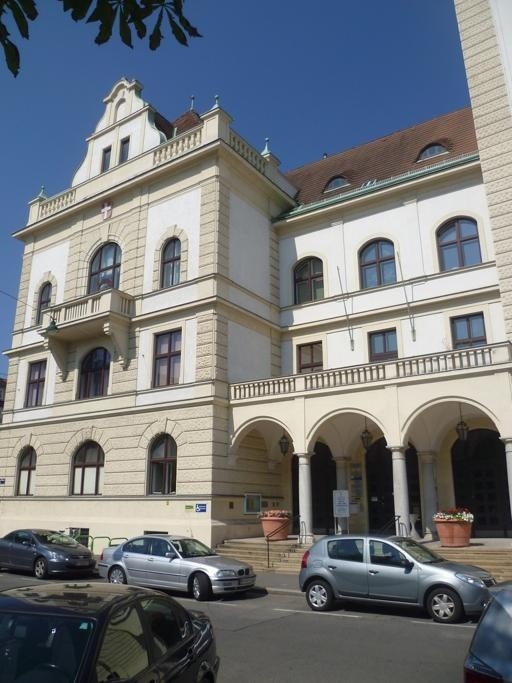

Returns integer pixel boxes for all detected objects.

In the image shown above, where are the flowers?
[256,508,291,520]
[431,507,475,523]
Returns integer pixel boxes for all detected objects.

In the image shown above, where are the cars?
[97,532,257,600]
[298,532,497,624]
[0,527,97,579]
[0,578,222,683]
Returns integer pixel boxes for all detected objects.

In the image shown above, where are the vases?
[433,518,471,548]
[260,516,290,541]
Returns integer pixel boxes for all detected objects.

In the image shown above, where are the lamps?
[46,315,59,334]
